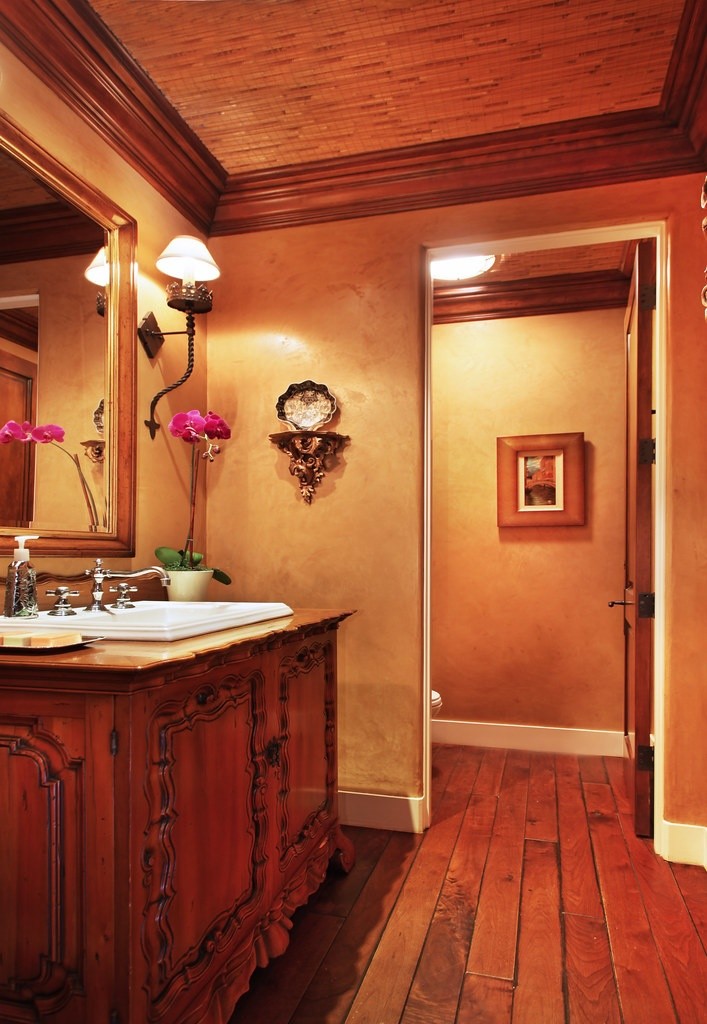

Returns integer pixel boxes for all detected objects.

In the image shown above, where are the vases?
[163,570,214,604]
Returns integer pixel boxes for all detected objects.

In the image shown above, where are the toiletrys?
[4,535,42,619]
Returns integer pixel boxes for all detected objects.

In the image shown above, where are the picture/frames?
[497,431,584,526]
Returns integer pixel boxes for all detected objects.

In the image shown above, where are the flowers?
[0,416,101,531]
[154,408,233,585]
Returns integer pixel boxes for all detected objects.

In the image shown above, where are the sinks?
[67,604,296,643]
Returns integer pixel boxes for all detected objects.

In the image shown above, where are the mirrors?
[0,108,138,556]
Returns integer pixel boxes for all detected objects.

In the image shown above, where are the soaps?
[0,631,82,648]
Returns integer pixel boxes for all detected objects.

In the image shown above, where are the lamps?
[84,247,110,431]
[138,234,220,437]
[428,250,505,281]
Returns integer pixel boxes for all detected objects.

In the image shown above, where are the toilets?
[431,690,444,720]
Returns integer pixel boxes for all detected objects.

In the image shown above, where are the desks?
[0,616,361,1024]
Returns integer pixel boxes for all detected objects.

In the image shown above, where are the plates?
[0,636,108,653]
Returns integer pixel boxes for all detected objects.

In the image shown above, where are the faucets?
[83,558,171,611]
